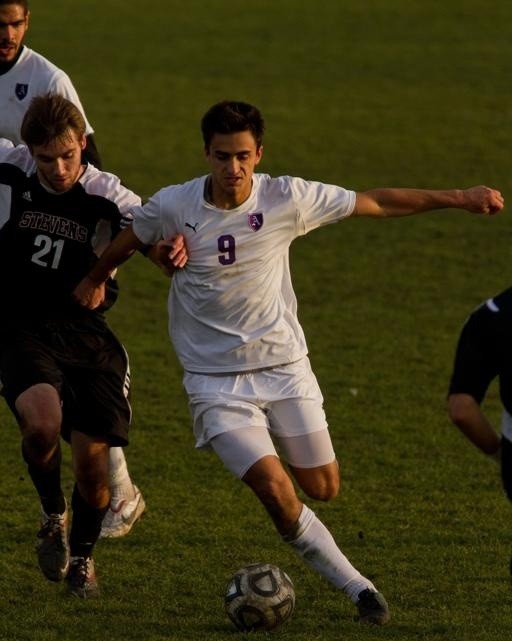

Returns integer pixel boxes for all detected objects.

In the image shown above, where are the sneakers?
[100,484,146,538]
[355,589,389,627]
[68,556,100,598]
[37,497,69,581]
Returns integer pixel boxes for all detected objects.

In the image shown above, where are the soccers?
[225,563,296,632]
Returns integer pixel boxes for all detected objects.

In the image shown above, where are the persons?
[0,0,145,537]
[0,96,187,598]
[71,102,505,628]
[447,288,511,503]
